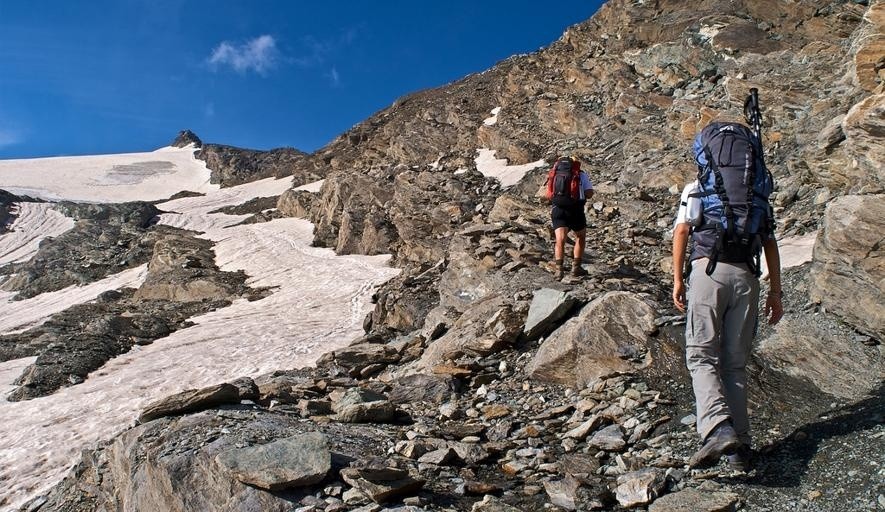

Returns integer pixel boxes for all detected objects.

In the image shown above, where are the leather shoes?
[728,443,753,471]
[690,420,741,469]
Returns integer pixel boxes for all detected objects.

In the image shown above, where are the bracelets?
[767,290,784,297]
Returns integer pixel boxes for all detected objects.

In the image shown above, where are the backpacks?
[693,122,773,263]
[546,156,581,208]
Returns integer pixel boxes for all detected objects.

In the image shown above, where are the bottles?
[684,184,703,227]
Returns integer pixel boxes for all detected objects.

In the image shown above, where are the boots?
[572,262,588,276]
[553,265,563,281]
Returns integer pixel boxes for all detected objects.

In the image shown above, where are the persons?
[550,155,594,283]
[670,180,786,480]
